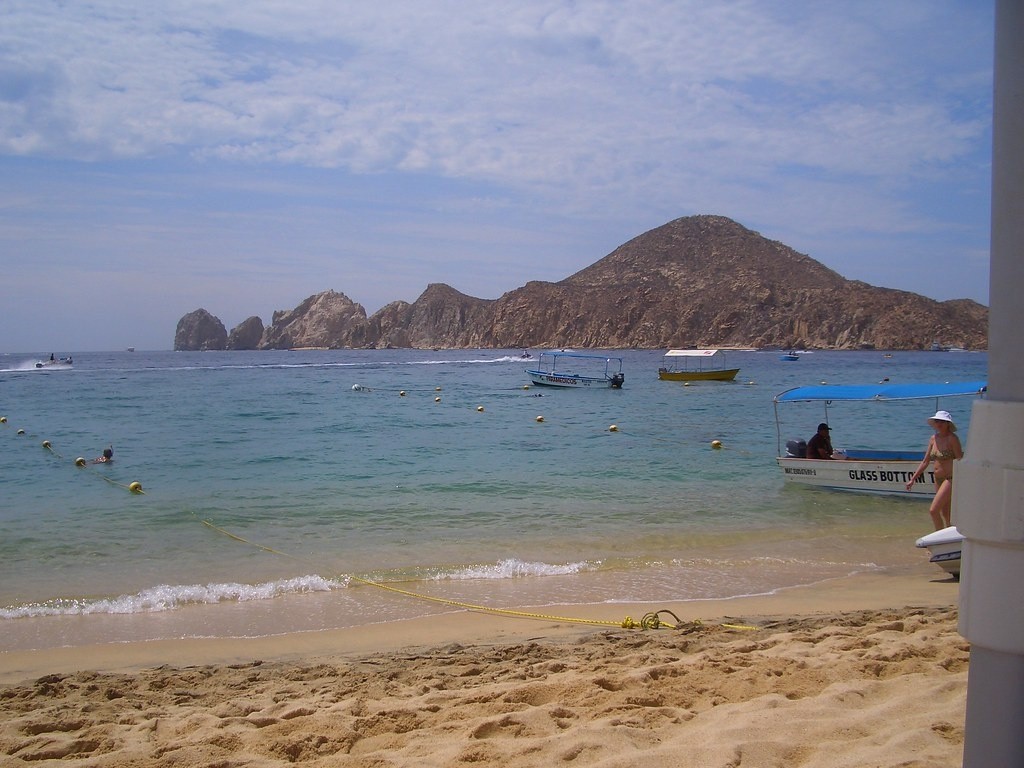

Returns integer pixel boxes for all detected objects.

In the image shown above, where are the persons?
[805,422,835,461]
[96,448,113,464]
[522,350,532,359]
[905,410,964,532]
[603,372,621,389]
[49,352,74,365]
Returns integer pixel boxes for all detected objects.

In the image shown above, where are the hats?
[928,411,957,432]
[818,423,832,430]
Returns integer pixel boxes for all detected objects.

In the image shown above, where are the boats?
[658,349,740,380]
[778,355,799,361]
[772,381,988,499]
[525,352,625,389]
[915,526,965,580]
[36,357,73,368]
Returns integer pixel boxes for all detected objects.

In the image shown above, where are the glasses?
[935,419,945,423]
